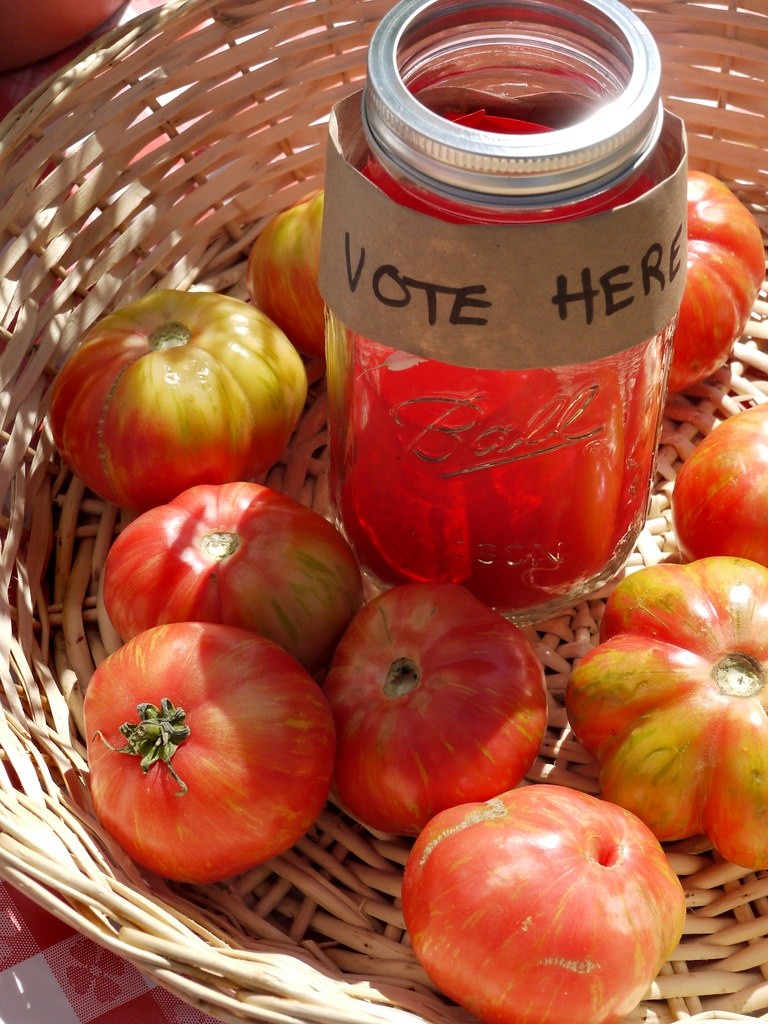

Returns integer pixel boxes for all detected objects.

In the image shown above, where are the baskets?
[0,0,765,1019]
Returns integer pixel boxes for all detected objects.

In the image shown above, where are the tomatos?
[47,156,764,1022]
